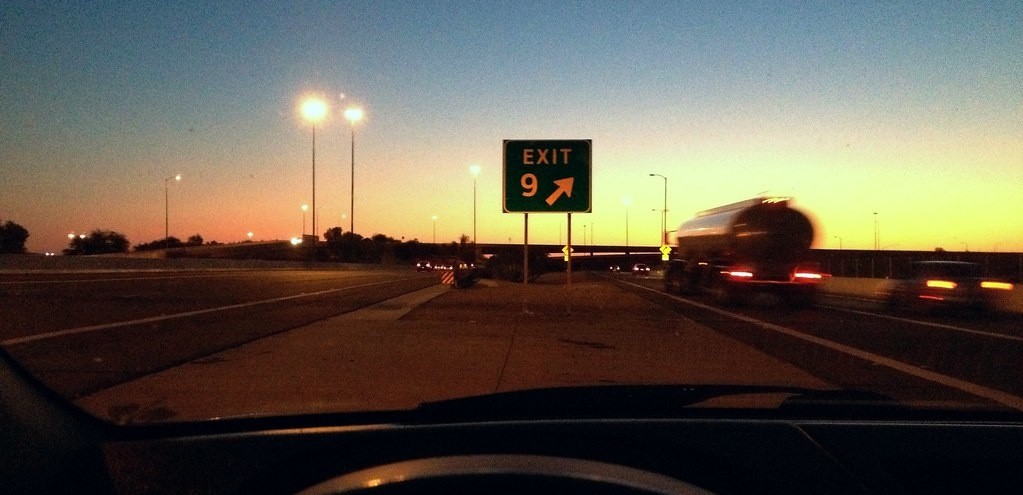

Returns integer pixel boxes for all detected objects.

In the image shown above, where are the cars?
[610,264,620,273]
[417,260,474,272]
[633,263,651,276]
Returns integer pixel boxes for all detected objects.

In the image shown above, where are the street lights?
[165,175,180,251]
[874,212,877,250]
[834,236,841,249]
[471,165,481,244]
[302,205,308,240]
[302,99,329,245]
[345,108,363,235]
[584,225,586,245]
[650,174,667,245]
[652,209,668,246]
[623,198,630,248]
[433,216,436,243]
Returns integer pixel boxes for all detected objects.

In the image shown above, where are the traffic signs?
[502,139,593,214]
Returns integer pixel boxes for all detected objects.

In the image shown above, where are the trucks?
[663,194,815,306]
